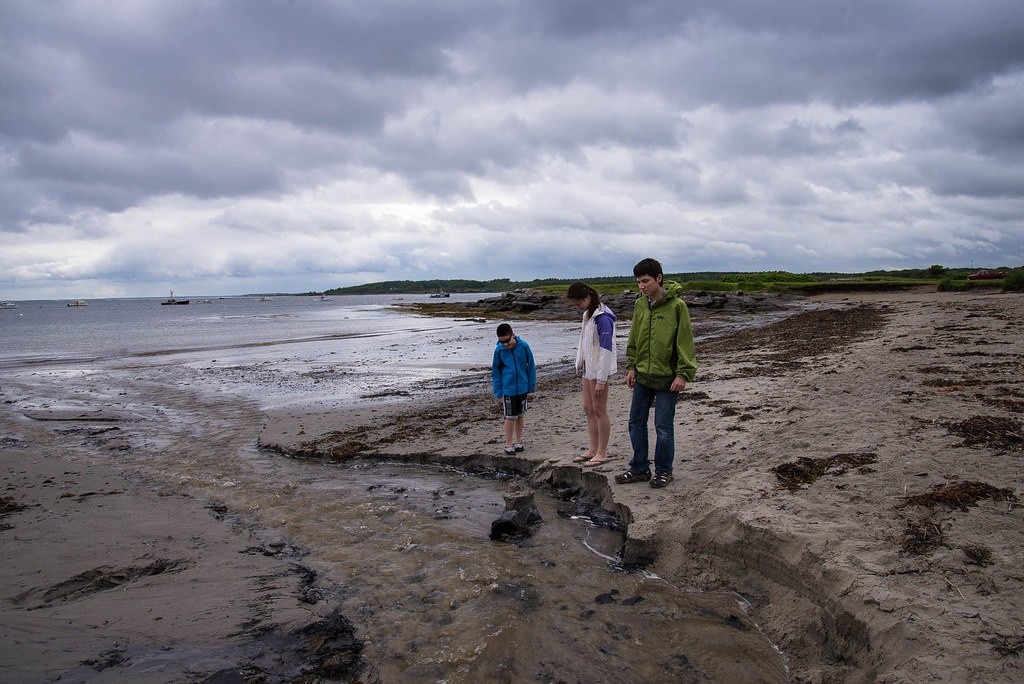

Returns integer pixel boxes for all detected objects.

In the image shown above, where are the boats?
[161,289,190,306]
[0,301,17,309]
[66,303,87,306]
[430,291,450,298]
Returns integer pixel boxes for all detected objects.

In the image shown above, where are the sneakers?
[650,471,673,488]
[616,467,652,484]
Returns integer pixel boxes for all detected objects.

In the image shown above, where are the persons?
[614,259,698,488]
[492,324,535,455]
[567,283,618,467]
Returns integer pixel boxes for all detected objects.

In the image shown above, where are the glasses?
[499,335,511,343]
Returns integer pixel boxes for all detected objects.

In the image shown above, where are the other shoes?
[505,446,515,455]
[514,443,524,452]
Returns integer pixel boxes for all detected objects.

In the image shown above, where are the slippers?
[584,459,610,467]
[573,455,592,462]
